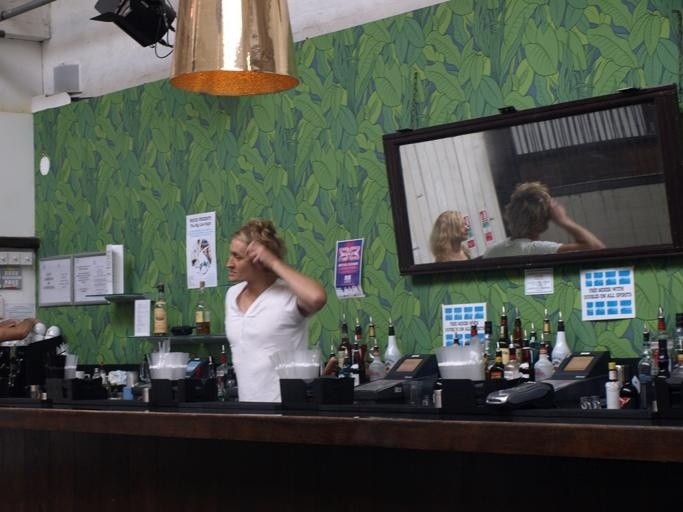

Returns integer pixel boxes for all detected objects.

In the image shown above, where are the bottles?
[91,368,110,393]
[463,209,495,259]
[326,312,400,388]
[206,344,236,401]
[194,280,210,335]
[603,306,682,410]
[28,384,48,408]
[449,303,571,389]
[152,281,169,338]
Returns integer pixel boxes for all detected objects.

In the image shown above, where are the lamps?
[88,1,301,97]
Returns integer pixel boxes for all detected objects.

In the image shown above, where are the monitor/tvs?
[551,351,611,380]
[385,355,441,380]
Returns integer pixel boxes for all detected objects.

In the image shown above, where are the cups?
[74,371,91,381]
[1,323,58,347]
[401,380,442,409]
[121,386,134,400]
[580,395,601,409]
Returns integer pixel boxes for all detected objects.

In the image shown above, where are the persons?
[430,210,472,261]
[481,181,609,262]
[0,313,39,346]
[223,220,329,404]
